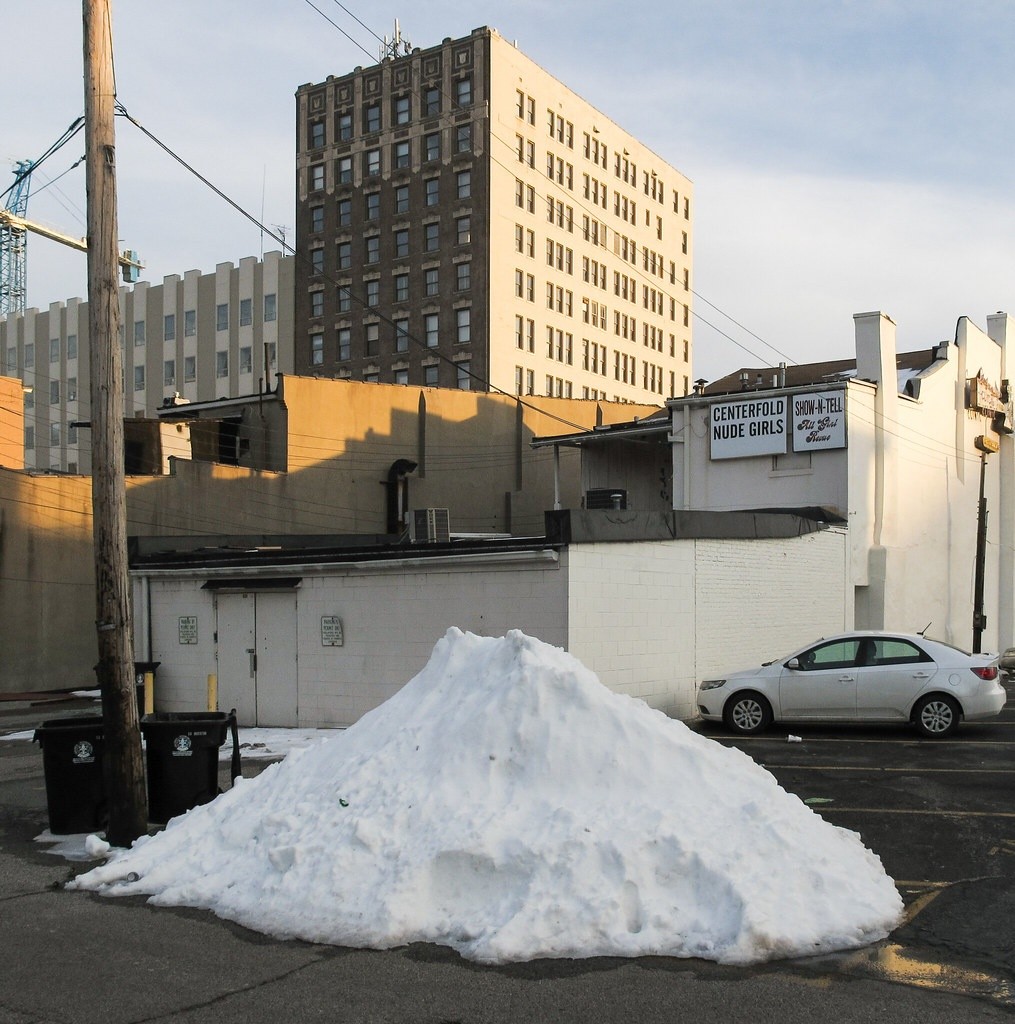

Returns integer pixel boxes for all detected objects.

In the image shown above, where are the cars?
[695,627,1007,742]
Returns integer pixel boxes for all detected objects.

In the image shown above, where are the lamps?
[739,373,749,388]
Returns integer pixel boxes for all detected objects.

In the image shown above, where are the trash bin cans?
[139,708,242,823]
[32,714,107,837]
[92,662,160,727]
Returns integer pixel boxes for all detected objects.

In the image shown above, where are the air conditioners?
[409,508,451,545]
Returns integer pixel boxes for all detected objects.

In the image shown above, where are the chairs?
[862,641,876,667]
